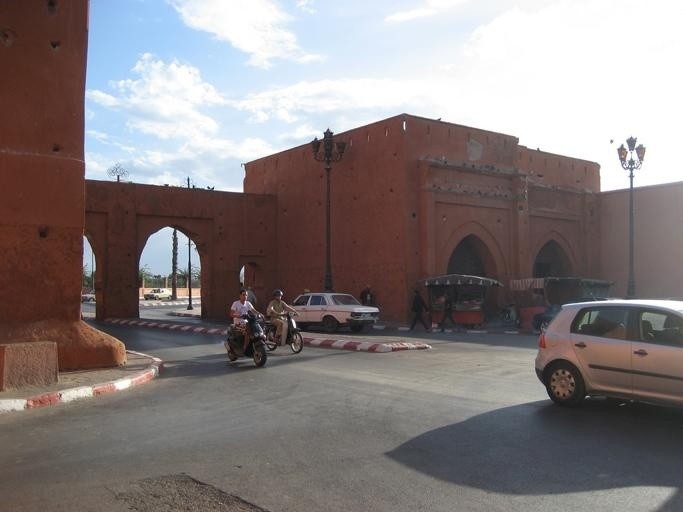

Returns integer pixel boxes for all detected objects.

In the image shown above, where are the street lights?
[311,126,346,291]
[616,135,647,300]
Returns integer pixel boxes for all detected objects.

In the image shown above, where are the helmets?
[271,290,283,296]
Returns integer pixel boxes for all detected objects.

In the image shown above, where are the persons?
[439,291,453,333]
[268,288,301,345]
[406,289,433,334]
[359,283,376,331]
[229,287,264,353]
[245,285,258,310]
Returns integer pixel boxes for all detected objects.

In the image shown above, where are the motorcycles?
[223,312,268,367]
[261,311,305,353]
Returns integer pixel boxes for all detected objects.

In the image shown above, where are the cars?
[143,288,172,300]
[282,293,380,333]
[534,298,682,410]
